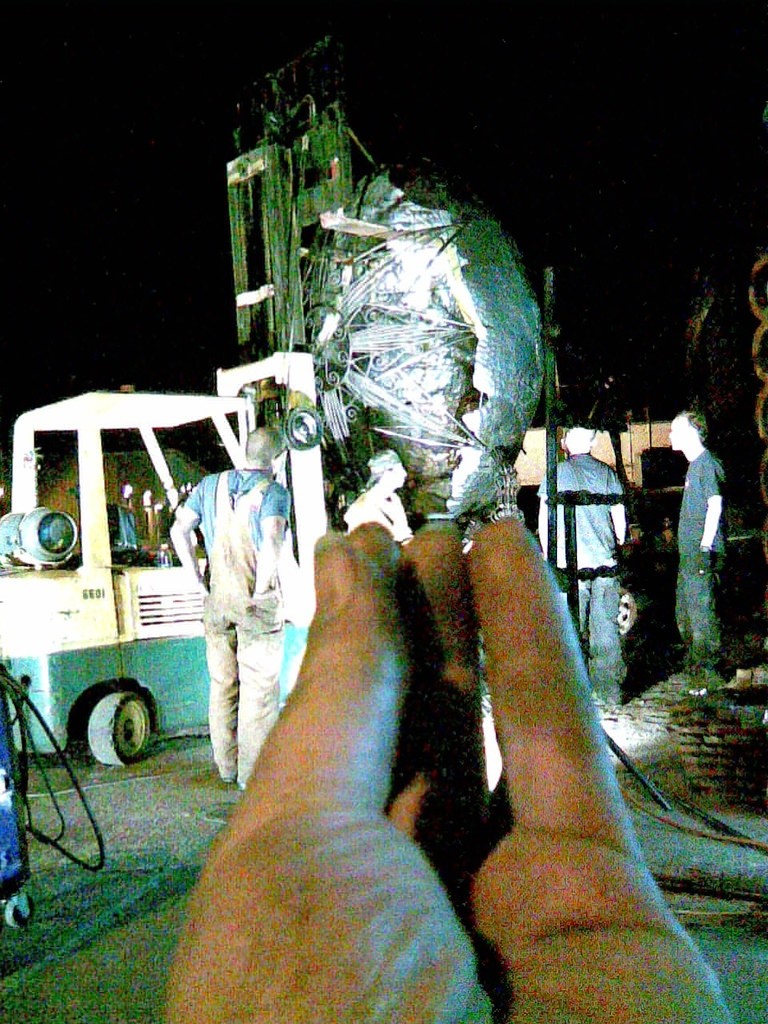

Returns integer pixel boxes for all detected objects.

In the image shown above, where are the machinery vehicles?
[0,51,359,765]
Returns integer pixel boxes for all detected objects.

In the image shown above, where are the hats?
[366,449,400,486]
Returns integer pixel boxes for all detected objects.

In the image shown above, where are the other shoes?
[219,766,236,782]
[237,779,246,790]
[680,672,725,696]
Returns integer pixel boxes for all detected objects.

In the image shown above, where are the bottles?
[116,481,209,568]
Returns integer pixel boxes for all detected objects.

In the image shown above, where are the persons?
[537,427,629,708]
[343,450,414,545]
[170,516,733,1024]
[669,412,727,696]
[170,426,291,793]
